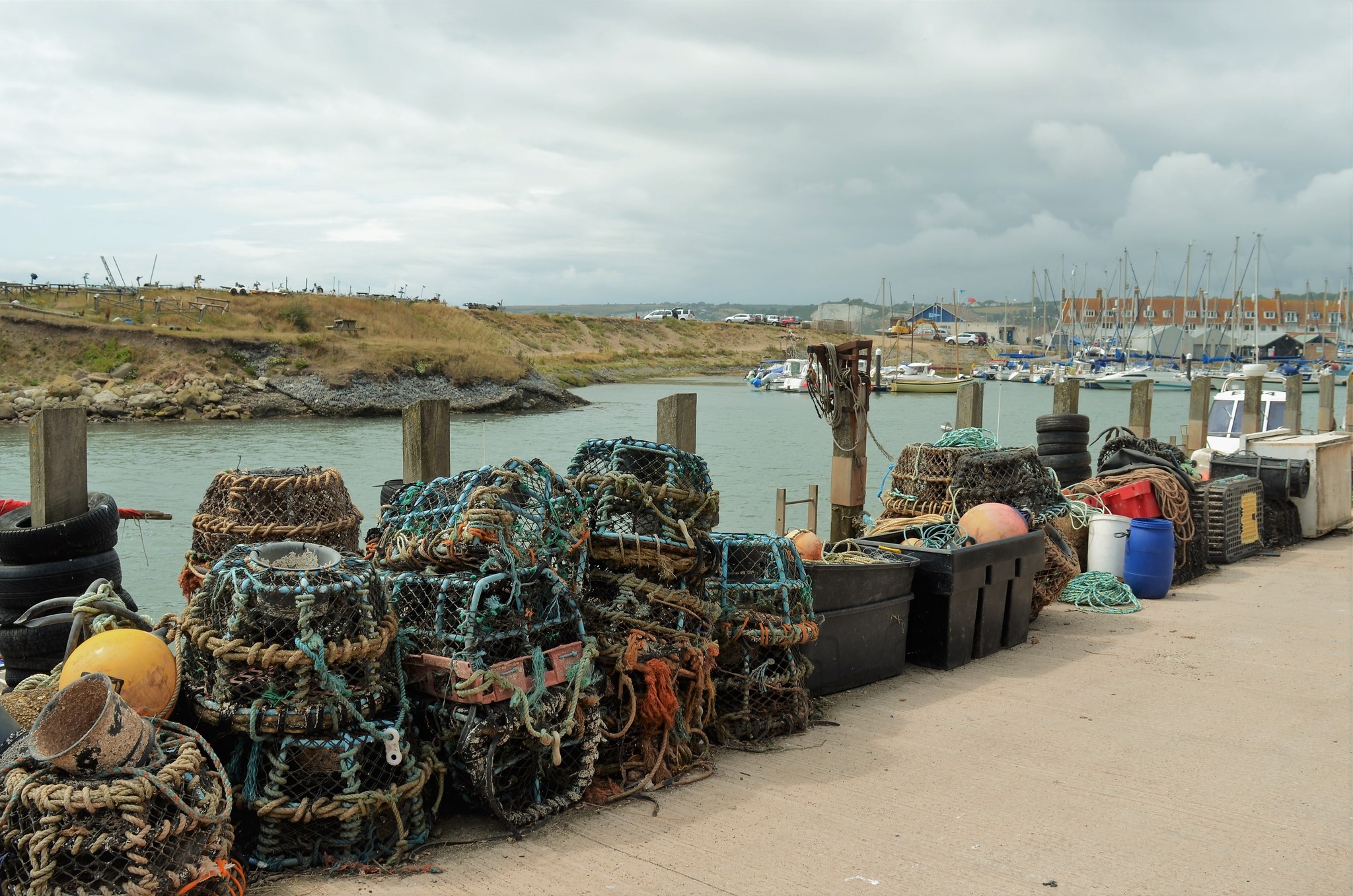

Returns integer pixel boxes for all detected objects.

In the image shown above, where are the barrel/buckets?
[982,372,988,378]
[753,379,761,388]
[1114,518,1174,600]
[1037,378,1041,384]
[750,378,755,384]
[749,375,754,380]
[250,542,341,618]
[1087,514,1132,582]
[28,673,155,779]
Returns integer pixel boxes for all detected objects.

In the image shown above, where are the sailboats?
[970,232,1353,394]
[845,276,976,393]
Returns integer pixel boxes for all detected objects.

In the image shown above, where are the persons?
[662,309,667,319]
[1026,336,1030,344]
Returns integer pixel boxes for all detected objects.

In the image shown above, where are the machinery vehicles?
[890,317,943,342]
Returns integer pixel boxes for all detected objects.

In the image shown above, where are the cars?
[928,328,952,338]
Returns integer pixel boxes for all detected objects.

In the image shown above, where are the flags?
[968,298,977,302]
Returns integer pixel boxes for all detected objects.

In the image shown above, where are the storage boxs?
[788,540,921,617]
[1084,479,1163,522]
[788,593,918,695]
[1204,429,1353,538]
[857,520,1046,671]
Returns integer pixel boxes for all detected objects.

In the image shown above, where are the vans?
[962,331,988,342]
[644,308,696,321]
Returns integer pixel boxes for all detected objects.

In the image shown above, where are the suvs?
[726,313,803,326]
[944,333,979,347]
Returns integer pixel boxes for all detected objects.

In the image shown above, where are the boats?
[1206,363,1289,455]
[744,358,867,393]
